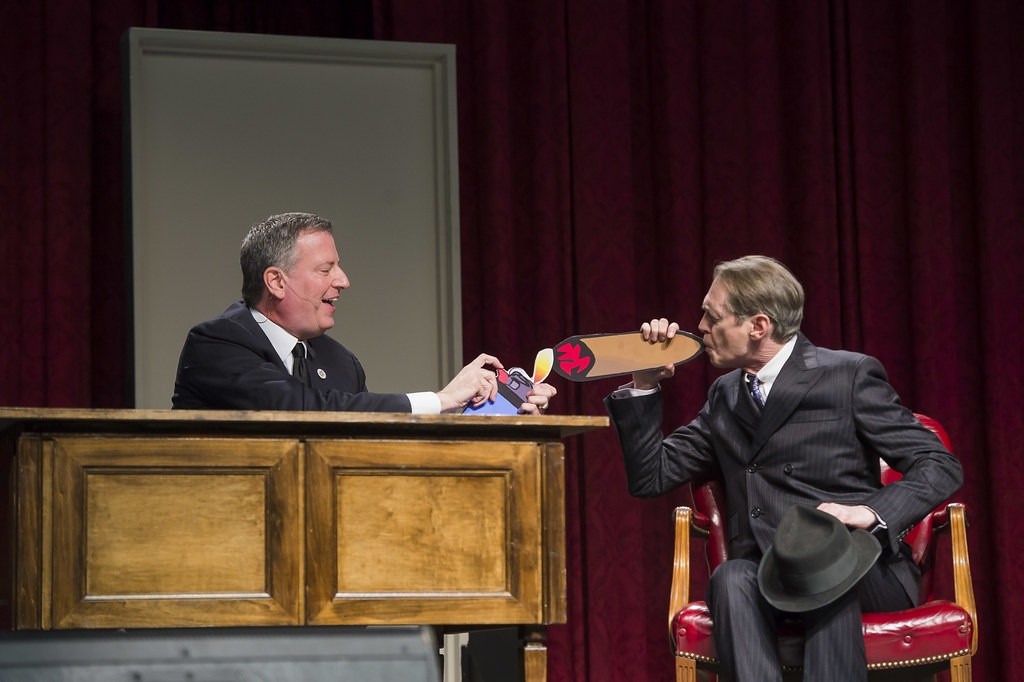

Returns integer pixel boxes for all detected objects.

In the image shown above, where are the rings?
[538,400,549,409]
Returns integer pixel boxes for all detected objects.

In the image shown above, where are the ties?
[749,376,765,411]
[291,343,309,387]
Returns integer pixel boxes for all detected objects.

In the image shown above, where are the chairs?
[667,414,976,682]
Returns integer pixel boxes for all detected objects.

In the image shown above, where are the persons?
[603,255,964,682]
[172,212,558,416]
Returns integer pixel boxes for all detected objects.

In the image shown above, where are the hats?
[757,504,882,612]
